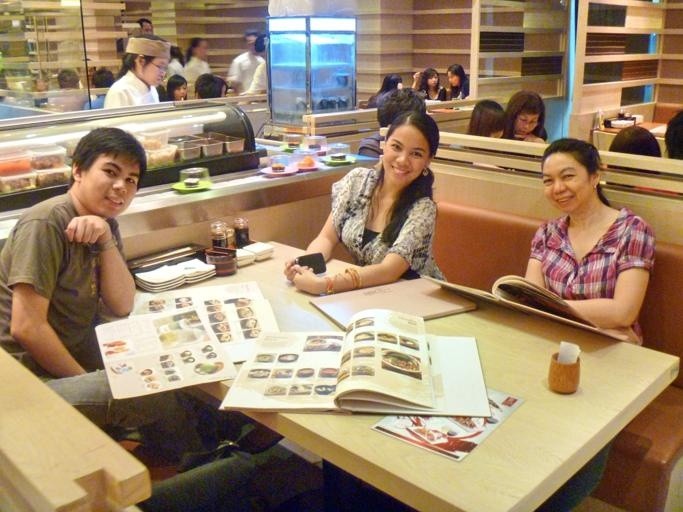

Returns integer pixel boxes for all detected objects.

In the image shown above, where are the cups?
[549,352,581,395]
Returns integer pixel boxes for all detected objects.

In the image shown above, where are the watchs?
[90,234,118,254]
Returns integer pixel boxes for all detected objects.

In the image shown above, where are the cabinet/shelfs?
[0,102,260,213]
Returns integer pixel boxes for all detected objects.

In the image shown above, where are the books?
[423,273,630,341]
[218,308,492,420]
[94,280,281,401]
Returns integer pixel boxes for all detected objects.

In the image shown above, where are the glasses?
[518,119,538,128]
[151,62,168,73]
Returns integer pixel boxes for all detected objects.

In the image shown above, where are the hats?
[125,34,171,58]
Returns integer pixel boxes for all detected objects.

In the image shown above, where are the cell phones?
[295,253,326,274]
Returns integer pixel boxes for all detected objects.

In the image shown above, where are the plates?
[236,248,255,267]
[323,157,357,166]
[289,162,318,171]
[177,259,217,284]
[135,266,185,292]
[243,242,274,261]
[171,181,211,193]
[261,167,298,177]
[382,350,422,374]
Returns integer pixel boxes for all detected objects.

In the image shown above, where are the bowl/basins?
[208,136,245,153]
[191,140,223,156]
[169,142,201,161]
[193,132,225,138]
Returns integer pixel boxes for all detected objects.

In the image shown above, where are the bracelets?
[326,268,362,293]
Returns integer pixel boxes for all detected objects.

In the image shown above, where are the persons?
[411,68,446,101]
[505,90,548,145]
[284,111,448,295]
[47,70,88,111]
[136,18,153,36]
[0,128,188,442]
[183,38,210,99]
[358,88,427,159]
[84,67,115,110]
[664,110,683,160]
[193,73,228,99]
[366,73,402,107]
[524,138,656,345]
[103,33,170,109]
[606,125,662,191]
[458,100,506,171]
[226,32,260,93]
[169,44,184,78]
[166,74,187,100]
[447,64,469,100]
[235,34,266,104]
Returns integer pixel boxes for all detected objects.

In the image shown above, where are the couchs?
[653,103,682,123]
[409,199,682,512]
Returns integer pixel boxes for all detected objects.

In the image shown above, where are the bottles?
[211,221,228,249]
[234,218,249,247]
[618,110,625,117]
